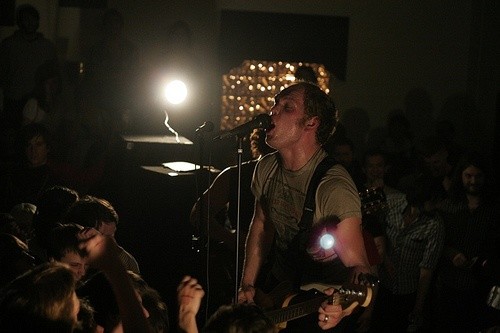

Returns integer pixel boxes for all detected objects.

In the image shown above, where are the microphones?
[195,121,214,133]
[211,114,271,143]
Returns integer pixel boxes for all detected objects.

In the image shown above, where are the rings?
[324,314,330,324]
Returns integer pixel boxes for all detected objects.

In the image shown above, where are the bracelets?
[239,282,256,291]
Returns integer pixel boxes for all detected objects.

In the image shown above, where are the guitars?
[229,272,380,333]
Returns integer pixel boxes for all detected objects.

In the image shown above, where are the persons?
[0,1,500,332]
[235,80,375,333]
[0,259,81,333]
[200,302,278,333]
